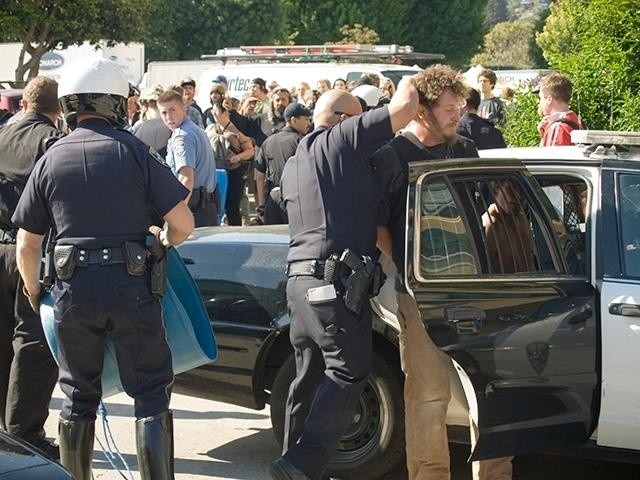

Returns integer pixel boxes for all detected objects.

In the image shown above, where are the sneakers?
[270,457,307,479]
[28,437,60,458]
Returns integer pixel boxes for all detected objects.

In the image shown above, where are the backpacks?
[210,131,241,170]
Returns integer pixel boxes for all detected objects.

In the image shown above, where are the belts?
[288,261,326,277]
[75,247,124,265]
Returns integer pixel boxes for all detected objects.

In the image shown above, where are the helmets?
[351,85,384,107]
[58,58,140,129]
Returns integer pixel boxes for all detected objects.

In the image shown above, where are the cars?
[0,427,78,480]
[1,79,26,116]
[145,125,640,480]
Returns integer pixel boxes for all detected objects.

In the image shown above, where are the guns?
[340,248,376,315]
[144,236,167,296]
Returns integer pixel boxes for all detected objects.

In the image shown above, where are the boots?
[136,411,173,479]
[58,414,94,479]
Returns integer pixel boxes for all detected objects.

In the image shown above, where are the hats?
[141,84,164,100]
[212,75,227,82]
[240,92,258,101]
[251,78,268,93]
[181,78,196,86]
[284,103,312,121]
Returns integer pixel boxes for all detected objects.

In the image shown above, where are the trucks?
[144,41,449,112]
